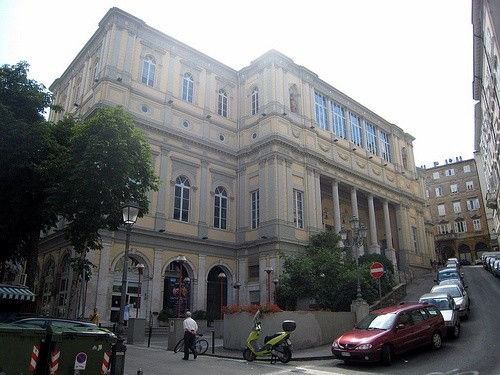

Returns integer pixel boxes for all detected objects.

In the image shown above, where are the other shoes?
[182,358,188,360]
[194,355,197,359]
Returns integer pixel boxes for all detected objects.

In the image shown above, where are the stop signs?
[371,262,384,279]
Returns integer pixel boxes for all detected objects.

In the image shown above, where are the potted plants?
[157,310,171,326]
[192,310,208,330]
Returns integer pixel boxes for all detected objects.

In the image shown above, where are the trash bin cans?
[43,325,117,375]
[0,324,47,375]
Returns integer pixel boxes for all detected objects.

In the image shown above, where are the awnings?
[0,287,37,302]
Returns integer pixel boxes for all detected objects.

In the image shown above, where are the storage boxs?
[282,320,296,331]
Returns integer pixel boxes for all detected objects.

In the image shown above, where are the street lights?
[134,262,145,318]
[176,254,186,318]
[233,282,243,306]
[264,266,273,305]
[110,196,144,375]
[220,273,226,320]
[184,277,190,316]
[272,279,279,291]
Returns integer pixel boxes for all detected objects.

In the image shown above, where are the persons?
[182,311,198,360]
[121,302,130,326]
[89,308,99,327]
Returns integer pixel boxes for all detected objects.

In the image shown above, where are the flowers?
[221,304,282,315]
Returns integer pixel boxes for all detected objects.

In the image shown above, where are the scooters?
[241,309,297,364]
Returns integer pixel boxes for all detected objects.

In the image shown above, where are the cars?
[418,257,471,339]
[331,300,445,368]
[9,316,98,330]
[475,251,500,279]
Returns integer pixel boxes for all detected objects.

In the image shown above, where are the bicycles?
[174,332,210,356]
